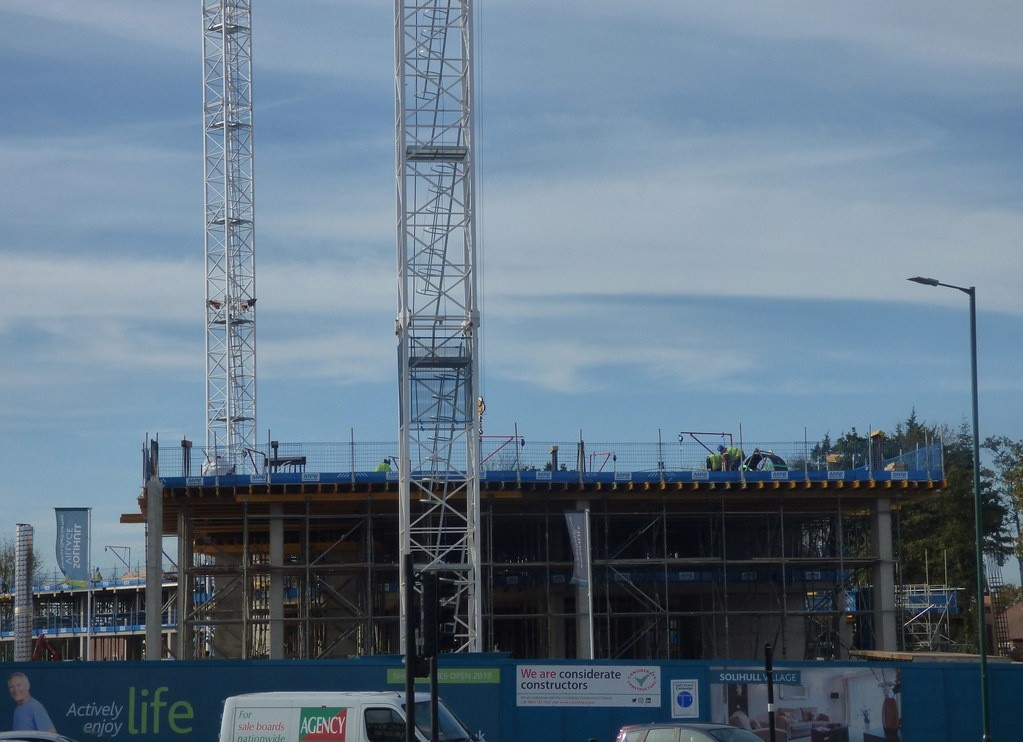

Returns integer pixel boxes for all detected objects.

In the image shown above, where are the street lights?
[905,276,992,742]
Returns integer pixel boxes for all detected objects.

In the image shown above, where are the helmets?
[382,458,391,463]
[717,445,724,451]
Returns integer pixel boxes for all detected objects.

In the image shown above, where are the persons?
[717,444,745,471]
[374,458,392,472]
[6,672,57,733]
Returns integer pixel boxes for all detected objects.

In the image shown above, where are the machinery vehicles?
[32,633,62,663]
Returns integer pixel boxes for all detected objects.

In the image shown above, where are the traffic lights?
[419,570,458,659]
[396,571,433,680]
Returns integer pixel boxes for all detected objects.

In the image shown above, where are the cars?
[615,719,767,742]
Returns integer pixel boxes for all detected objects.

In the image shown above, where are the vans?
[217,688,479,742]
